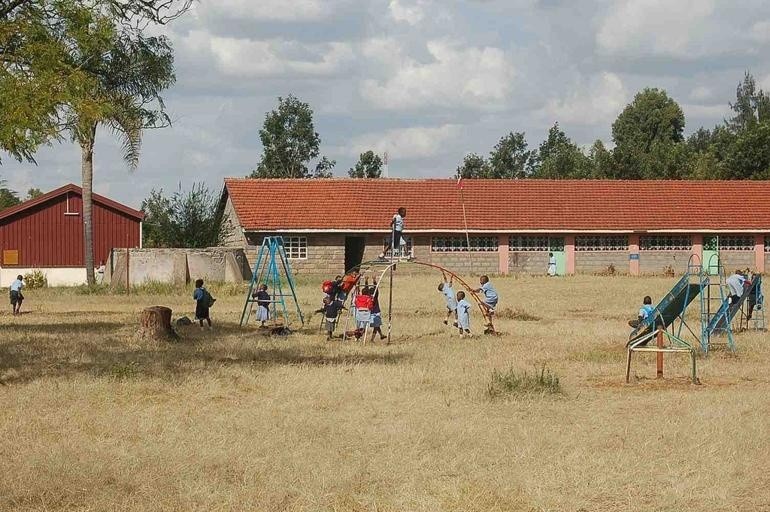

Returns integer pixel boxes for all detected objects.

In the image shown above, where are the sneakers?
[443,319,472,339]
[199,327,213,332]
[327,332,388,342]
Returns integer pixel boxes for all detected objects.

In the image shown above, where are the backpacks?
[201,291,216,309]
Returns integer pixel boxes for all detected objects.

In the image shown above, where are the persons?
[727,270,764,320]
[314,271,387,344]
[376,207,417,263]
[547,253,558,276]
[252,284,270,329]
[438,268,473,339]
[628,296,654,328]
[194,280,213,332]
[10,275,24,316]
[471,276,498,316]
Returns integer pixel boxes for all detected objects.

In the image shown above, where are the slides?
[706,273,760,334]
[626,274,709,347]
